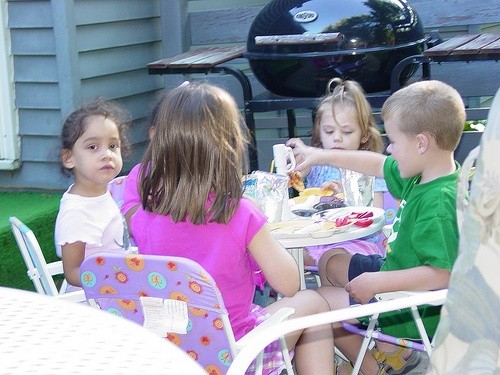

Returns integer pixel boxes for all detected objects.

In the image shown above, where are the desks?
[145,33,500,173]
[0,286,207,375]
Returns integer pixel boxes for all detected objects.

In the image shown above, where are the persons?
[122,77,471,375]
[54,97,130,295]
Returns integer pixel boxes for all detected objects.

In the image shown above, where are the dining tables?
[262,204,386,289]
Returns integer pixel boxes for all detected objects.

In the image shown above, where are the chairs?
[8,216,86,304]
[80,253,294,375]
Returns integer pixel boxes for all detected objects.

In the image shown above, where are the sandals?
[334,341,421,375]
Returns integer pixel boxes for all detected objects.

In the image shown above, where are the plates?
[270,218,356,239]
